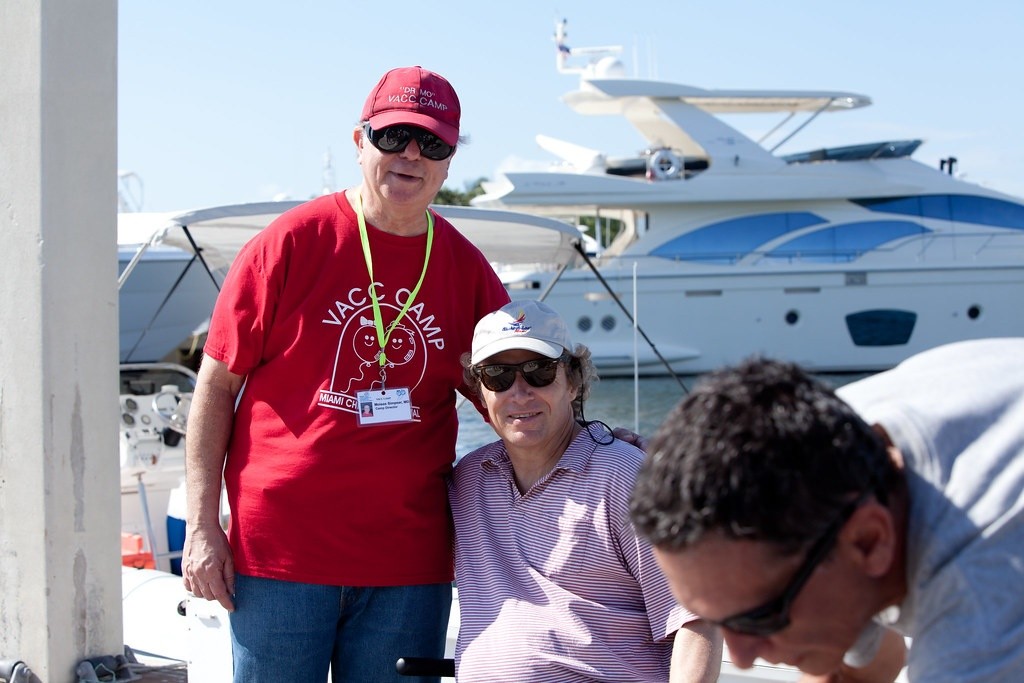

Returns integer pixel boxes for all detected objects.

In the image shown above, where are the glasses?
[471,356,574,393]
[365,121,456,162]
[709,478,885,638]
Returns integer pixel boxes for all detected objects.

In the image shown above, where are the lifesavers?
[651,149,683,180]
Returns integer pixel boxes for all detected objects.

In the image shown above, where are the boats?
[473,20,1024,376]
[117,195,697,578]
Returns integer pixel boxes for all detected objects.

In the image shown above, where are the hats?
[362,65,461,147]
[471,299,573,367]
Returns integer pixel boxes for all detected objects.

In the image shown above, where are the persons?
[362,404,372,417]
[181,64,648,683]
[628,337,1024,683]
[449,300,722,683]
